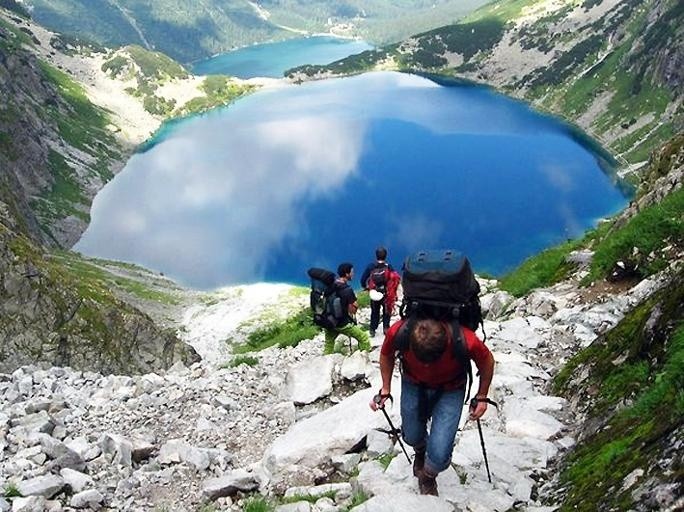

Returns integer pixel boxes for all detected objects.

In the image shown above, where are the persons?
[361,247,400,337]
[370,317,494,496]
[318,263,369,355]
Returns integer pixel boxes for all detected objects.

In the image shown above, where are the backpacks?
[307,267,353,332]
[367,262,389,302]
[393,247,484,370]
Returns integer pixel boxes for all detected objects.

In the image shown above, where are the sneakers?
[419,472,438,498]
[411,453,424,477]
